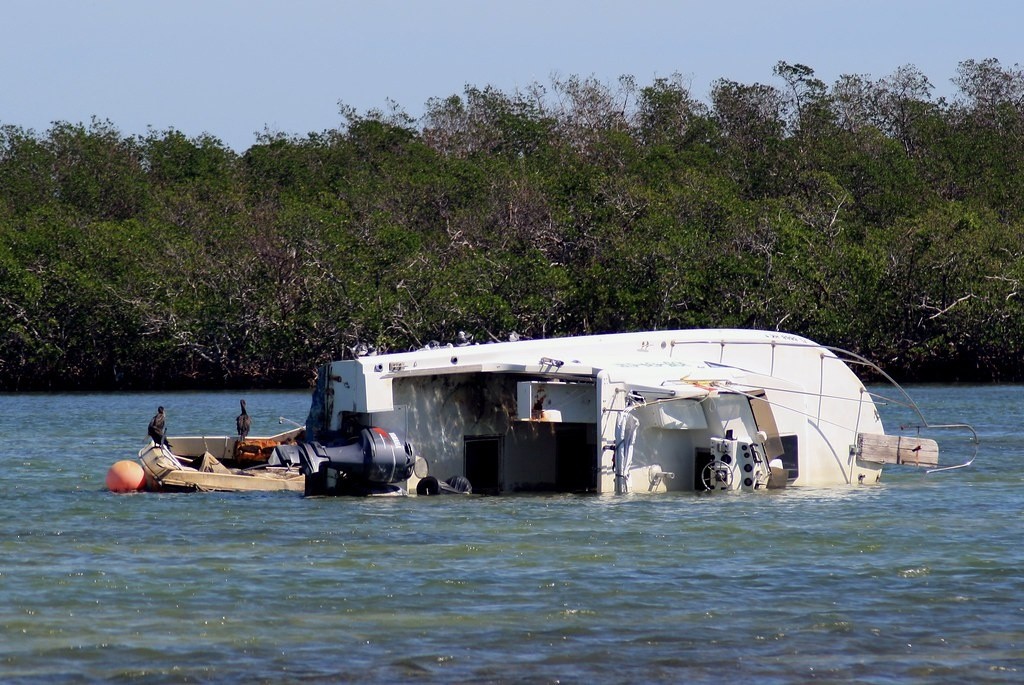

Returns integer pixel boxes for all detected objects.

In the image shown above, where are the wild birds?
[147,403,174,451]
[234,398,251,442]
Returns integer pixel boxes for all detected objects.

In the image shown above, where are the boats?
[104,415,310,495]
[272,323,977,502]
